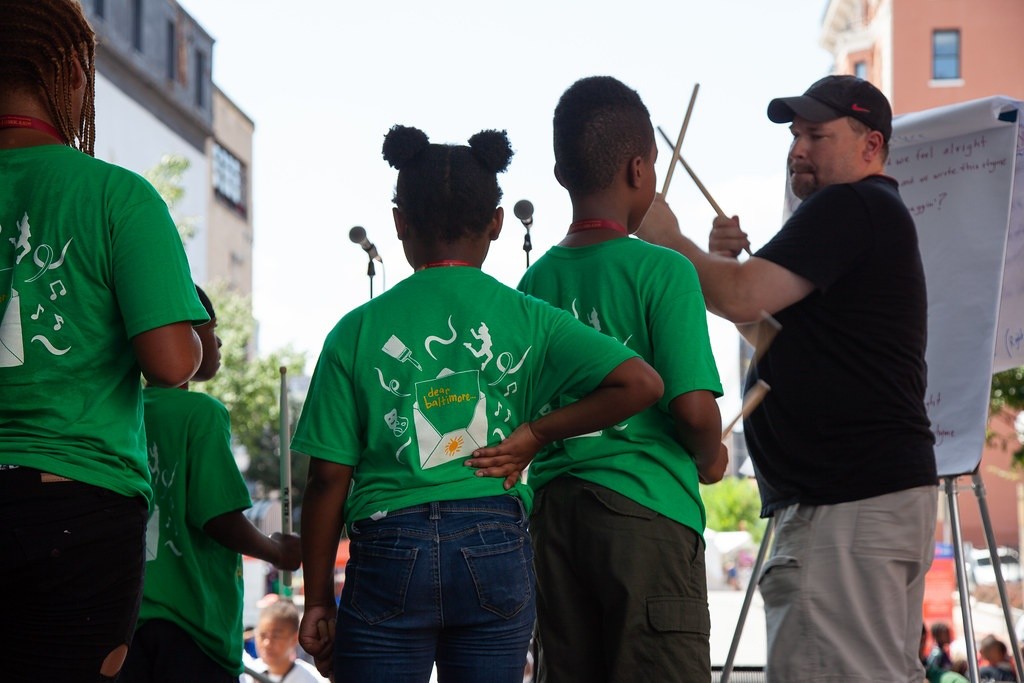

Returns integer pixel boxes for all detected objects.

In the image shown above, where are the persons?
[0,1,212,683]
[918,622,1018,683]
[633,75,942,683]
[238,593,332,683]
[518,78,753,683]
[125,285,304,683]
[299,124,663,683]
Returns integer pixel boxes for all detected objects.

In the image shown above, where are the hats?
[767,74,894,140]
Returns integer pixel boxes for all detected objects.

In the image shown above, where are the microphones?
[349,226,383,263]
[513,200,534,229]
